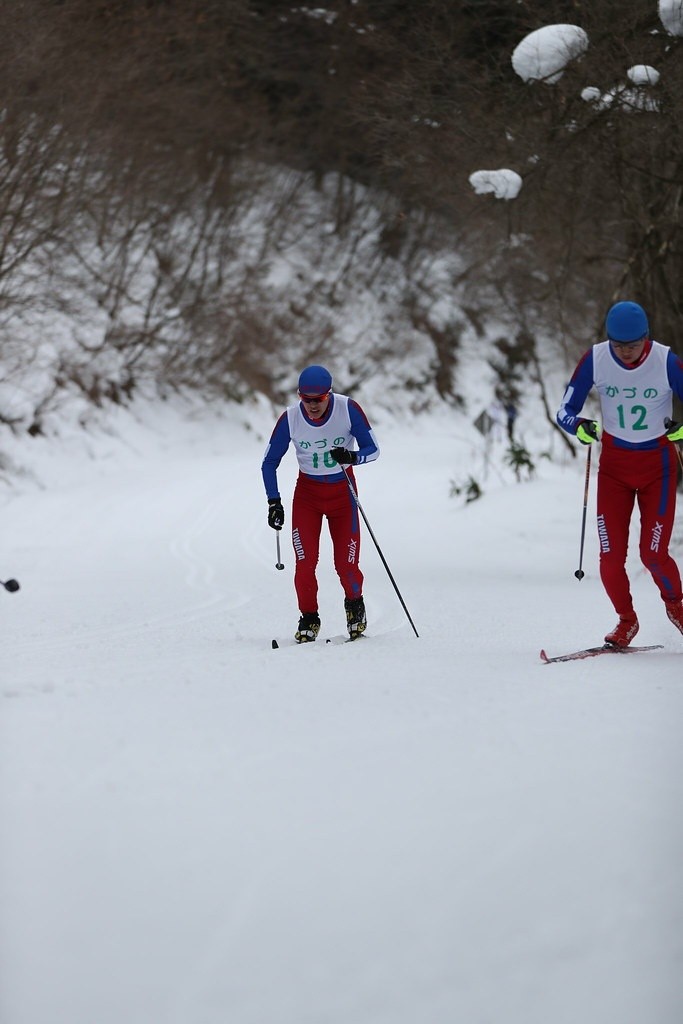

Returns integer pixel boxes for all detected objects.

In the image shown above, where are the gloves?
[268,499,284,530]
[576,420,599,445]
[330,447,357,464]
[666,422,683,441]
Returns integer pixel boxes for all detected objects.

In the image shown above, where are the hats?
[607,301,648,343]
[299,365,332,395]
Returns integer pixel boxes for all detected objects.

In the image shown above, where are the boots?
[296,612,320,642]
[345,597,366,633]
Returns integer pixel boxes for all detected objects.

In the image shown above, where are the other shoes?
[666,601,683,634]
[605,620,639,647]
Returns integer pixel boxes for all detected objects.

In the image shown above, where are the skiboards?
[271,639,331,649]
[538,643,666,663]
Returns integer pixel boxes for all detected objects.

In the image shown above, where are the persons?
[556,302,683,648]
[261,365,380,644]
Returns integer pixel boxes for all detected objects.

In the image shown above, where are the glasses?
[300,395,328,403]
[610,341,642,350]
